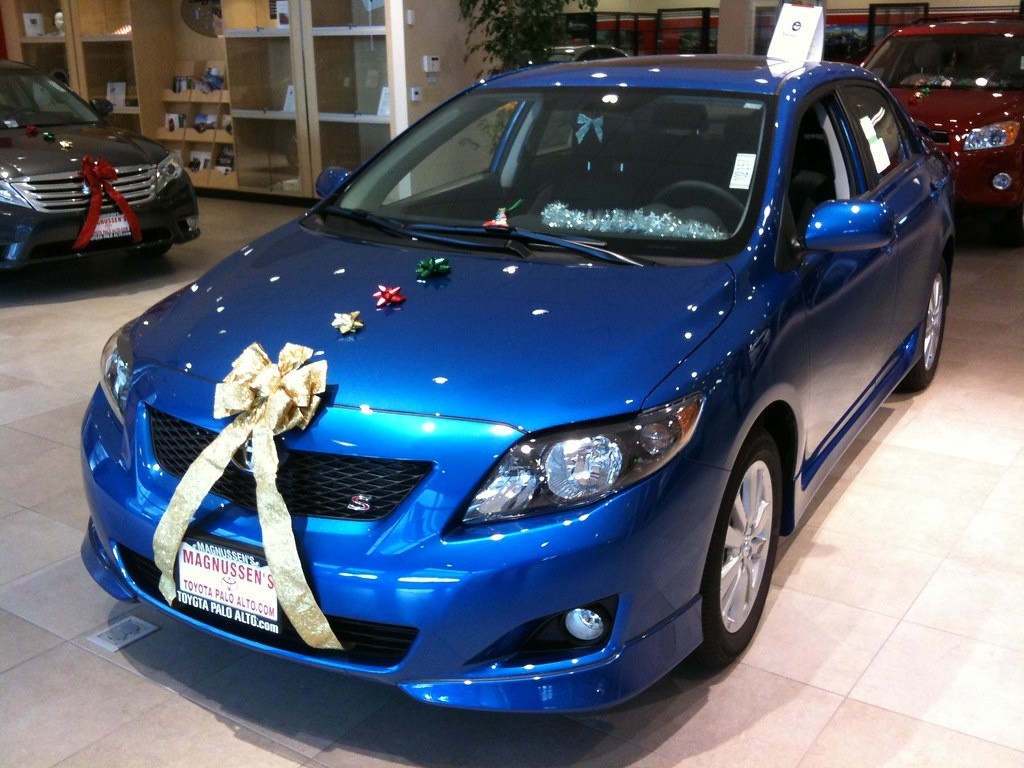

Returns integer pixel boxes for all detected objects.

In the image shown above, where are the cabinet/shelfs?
[0,0,390,199]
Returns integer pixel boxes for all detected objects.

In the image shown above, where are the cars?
[1,59,202,270]
[844,16,1024,236]
[79,52,956,714]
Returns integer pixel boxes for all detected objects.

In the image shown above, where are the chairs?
[526,104,847,235]
[898,41,945,85]
